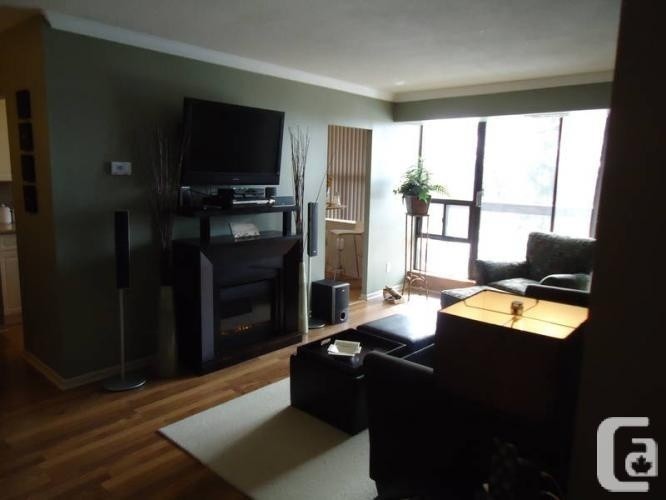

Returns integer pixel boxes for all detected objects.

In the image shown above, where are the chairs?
[474,232,597,296]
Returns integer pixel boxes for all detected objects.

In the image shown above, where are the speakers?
[308,202,318,256]
[112,210,129,288]
[313,279,350,324]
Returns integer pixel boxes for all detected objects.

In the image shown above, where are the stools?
[328,228,363,280]
[441,285,515,310]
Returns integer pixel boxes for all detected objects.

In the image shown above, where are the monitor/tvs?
[175,95,285,197]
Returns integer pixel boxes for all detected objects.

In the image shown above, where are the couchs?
[364,352,579,500]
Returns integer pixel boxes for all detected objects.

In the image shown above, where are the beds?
[401,213,429,301]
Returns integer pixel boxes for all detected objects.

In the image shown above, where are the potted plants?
[394,158,450,214]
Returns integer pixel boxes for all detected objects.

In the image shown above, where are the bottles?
[384,284,401,300]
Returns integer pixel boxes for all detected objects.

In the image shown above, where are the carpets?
[158,376,380,500]
